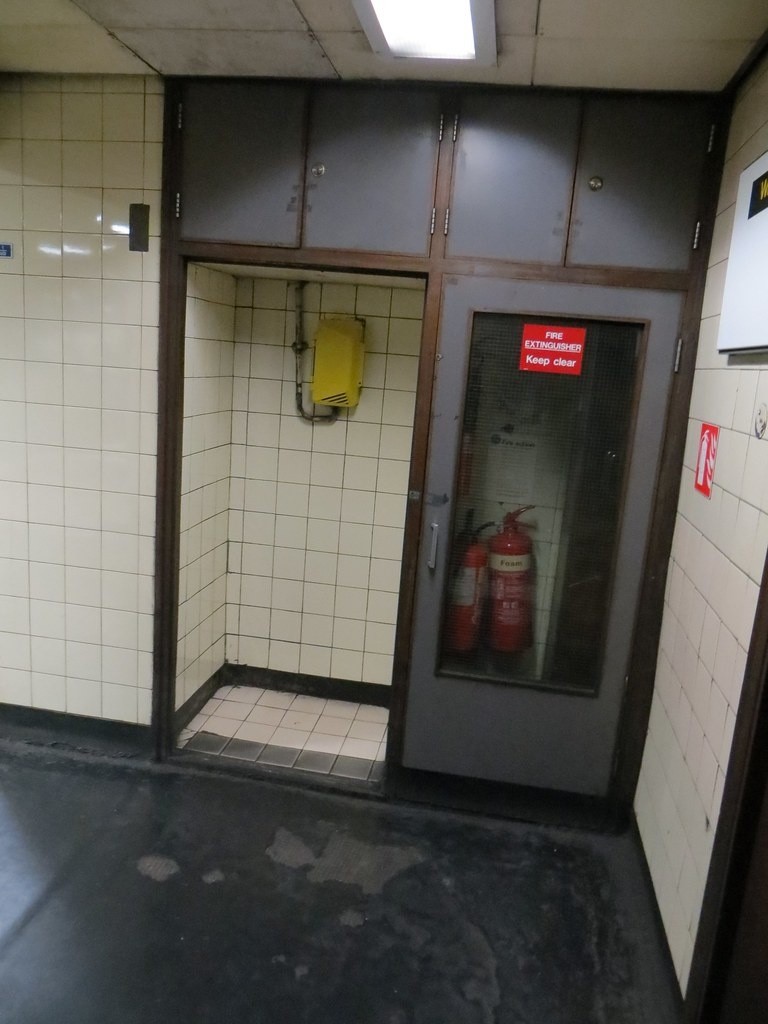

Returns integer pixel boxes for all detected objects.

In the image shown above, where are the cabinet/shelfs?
[161,75,729,271]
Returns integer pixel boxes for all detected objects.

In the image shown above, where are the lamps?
[352,0,497,65]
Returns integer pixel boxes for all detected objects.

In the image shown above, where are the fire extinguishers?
[445,509,495,656]
[489,503,541,654]
